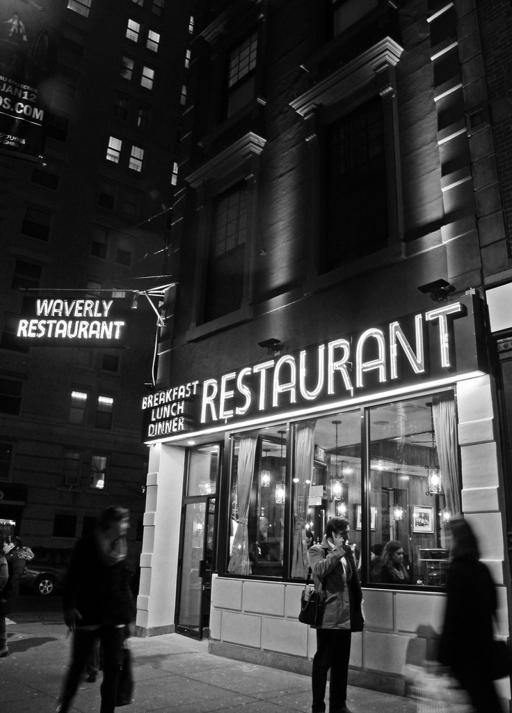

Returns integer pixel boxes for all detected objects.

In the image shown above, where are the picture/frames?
[411,504,435,535]
[353,503,377,532]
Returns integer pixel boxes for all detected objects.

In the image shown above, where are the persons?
[55,505,141,712]
[429,518,504,713]
[345,539,426,586]
[1,534,34,658]
[307,517,366,713]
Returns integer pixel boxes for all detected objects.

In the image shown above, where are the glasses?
[397,551,405,555]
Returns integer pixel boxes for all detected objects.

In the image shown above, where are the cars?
[20,554,65,598]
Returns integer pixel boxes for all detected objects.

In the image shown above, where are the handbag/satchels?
[298,589,326,626]
[494,634,511,680]
[113,640,136,707]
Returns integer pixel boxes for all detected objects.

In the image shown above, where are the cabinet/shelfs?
[255,459,284,567]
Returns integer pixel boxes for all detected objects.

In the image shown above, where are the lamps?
[394,468,403,523]
[424,402,442,495]
[331,420,344,502]
[257,337,281,353]
[417,279,456,301]
[130,290,165,328]
[260,430,287,506]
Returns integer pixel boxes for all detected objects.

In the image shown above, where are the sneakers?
[84,670,98,682]
[0,645,9,657]
